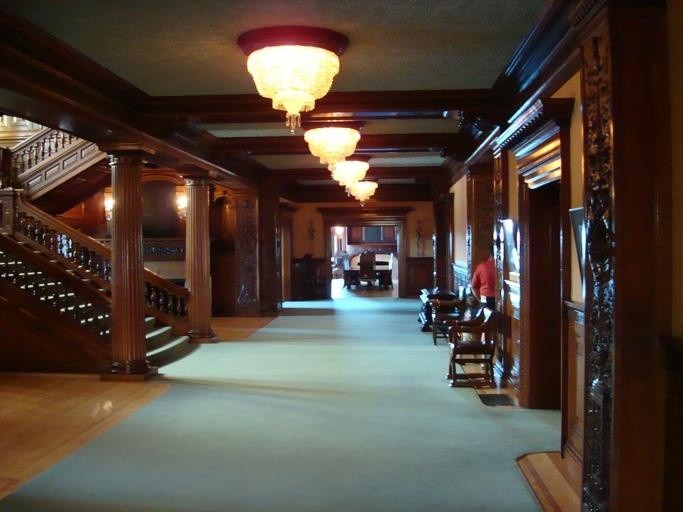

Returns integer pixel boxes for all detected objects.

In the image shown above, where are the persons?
[471,248,496,312]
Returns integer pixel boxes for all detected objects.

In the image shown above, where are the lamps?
[236,25,380,209]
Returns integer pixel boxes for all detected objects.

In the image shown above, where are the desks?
[418,286,456,333]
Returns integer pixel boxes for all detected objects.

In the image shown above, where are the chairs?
[341,250,394,290]
[428,291,499,387]
